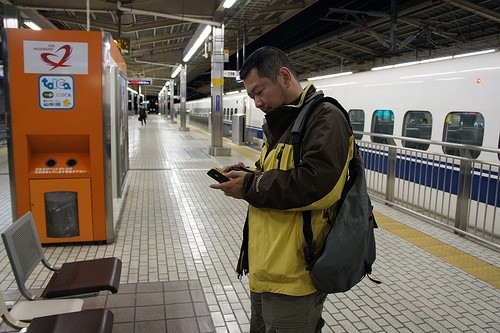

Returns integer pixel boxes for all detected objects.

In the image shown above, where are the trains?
[173,48,500,239]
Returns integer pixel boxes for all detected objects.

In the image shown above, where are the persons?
[210,46,353,333]
[139,103,147,126]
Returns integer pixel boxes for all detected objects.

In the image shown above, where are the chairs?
[0,293,114,333]
[1,212,122,299]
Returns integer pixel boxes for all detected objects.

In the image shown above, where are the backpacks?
[290,97,381,303]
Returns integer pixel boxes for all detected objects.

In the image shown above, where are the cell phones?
[207,169,230,184]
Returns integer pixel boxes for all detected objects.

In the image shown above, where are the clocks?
[113,38,131,56]
